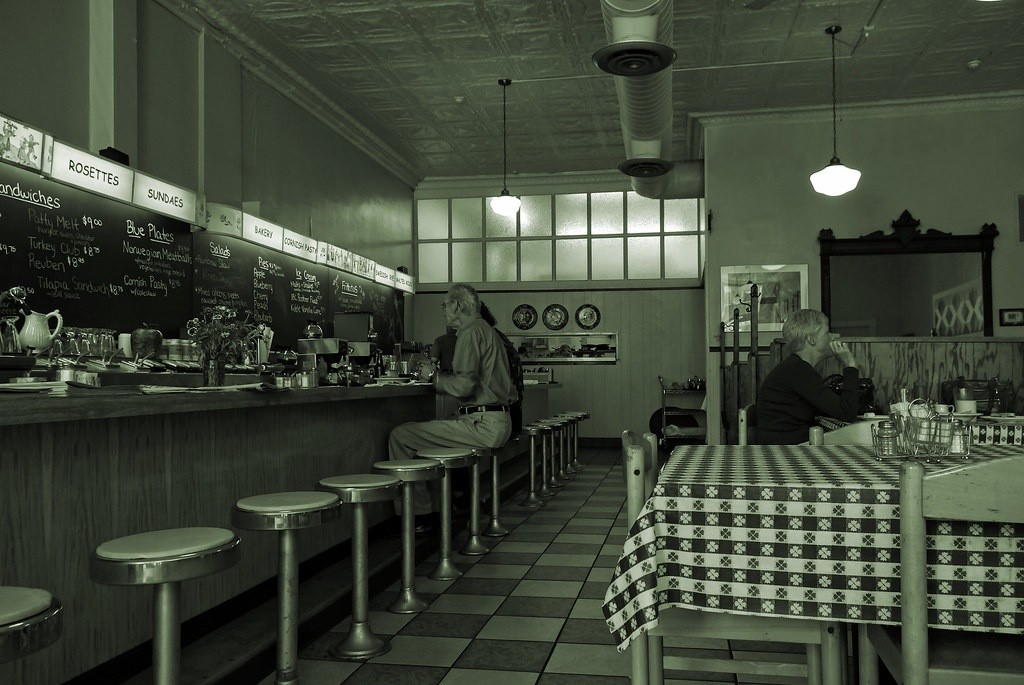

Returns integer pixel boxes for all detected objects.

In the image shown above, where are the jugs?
[302,319,326,339]
[275,345,297,364]
[18,308,62,352]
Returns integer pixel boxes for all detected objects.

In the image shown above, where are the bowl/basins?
[939,413,983,423]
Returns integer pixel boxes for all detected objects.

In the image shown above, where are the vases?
[201,358,227,387]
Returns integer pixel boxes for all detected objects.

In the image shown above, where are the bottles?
[914,379,927,404]
[56,362,75,383]
[878,421,897,456]
[156,338,199,361]
[275,366,319,389]
[374,354,385,377]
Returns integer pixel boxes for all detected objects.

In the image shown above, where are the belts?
[459,404,508,415]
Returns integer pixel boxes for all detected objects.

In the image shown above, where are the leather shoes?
[391,515,440,537]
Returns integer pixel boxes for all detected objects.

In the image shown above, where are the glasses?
[441,301,461,311]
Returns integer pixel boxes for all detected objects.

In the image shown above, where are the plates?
[980,415,1024,423]
[373,377,410,383]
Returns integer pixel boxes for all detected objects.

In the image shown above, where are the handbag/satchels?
[824,373,875,415]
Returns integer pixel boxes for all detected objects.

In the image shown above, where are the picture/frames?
[721,263,808,332]
[575,304,601,330]
[542,304,569,330]
[512,304,538,330]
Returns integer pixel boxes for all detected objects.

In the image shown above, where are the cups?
[956,400,978,422]
[48,327,117,357]
[894,388,913,402]
[118,333,132,358]
[2,316,22,353]
[935,404,954,414]
[808,426,823,445]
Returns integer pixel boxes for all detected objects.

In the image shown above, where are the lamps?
[490,77,521,217]
[809,26,861,198]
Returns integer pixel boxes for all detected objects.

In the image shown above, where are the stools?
[0,584,65,665]
[89,447,477,685]
[458,409,591,554]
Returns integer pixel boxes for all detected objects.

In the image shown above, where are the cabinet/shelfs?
[658,375,706,448]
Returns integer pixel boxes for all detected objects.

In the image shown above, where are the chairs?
[621,404,1024,685]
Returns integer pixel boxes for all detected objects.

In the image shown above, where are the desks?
[601,443,1024,685]
[820,415,1024,444]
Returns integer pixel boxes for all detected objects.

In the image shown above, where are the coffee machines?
[297,338,348,388]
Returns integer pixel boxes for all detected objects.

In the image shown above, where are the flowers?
[185,304,270,372]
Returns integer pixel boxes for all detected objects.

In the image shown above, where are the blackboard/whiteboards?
[0,161,192,344]
[192,230,404,354]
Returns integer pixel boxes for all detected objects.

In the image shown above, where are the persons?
[753,309,861,446]
[389,283,525,535]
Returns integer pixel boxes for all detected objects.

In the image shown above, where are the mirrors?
[818,208,1001,338]
